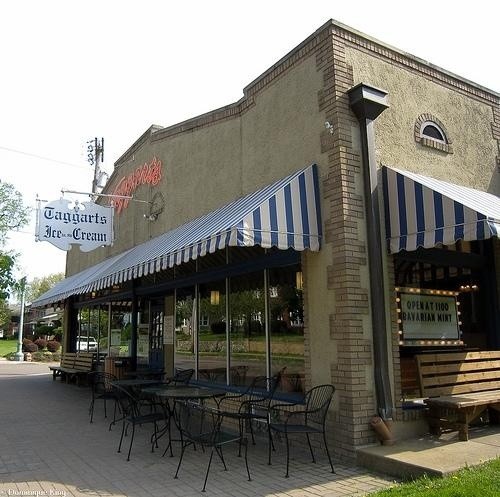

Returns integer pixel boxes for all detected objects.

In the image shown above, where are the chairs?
[87,366,335,492]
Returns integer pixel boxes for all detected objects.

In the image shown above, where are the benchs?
[48,352,93,387]
[415,349,500,441]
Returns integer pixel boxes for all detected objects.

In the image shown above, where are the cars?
[75,336,100,352]
